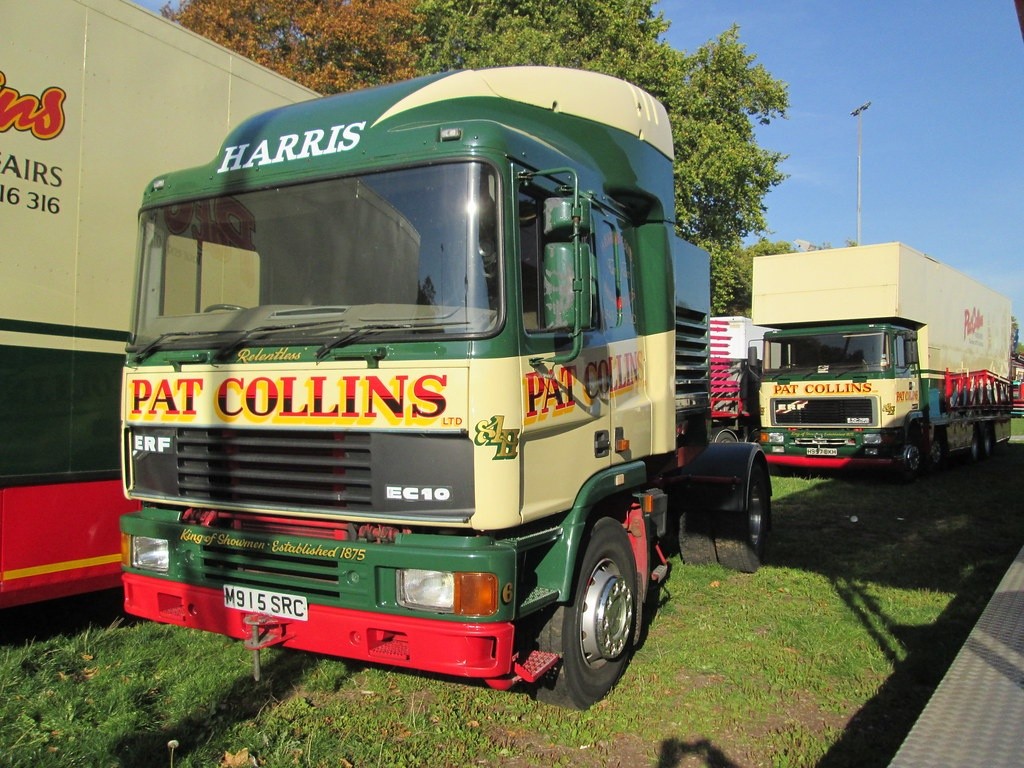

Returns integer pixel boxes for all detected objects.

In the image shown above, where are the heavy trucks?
[745,238,1017,475]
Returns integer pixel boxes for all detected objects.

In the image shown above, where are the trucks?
[115,60,771,712]
